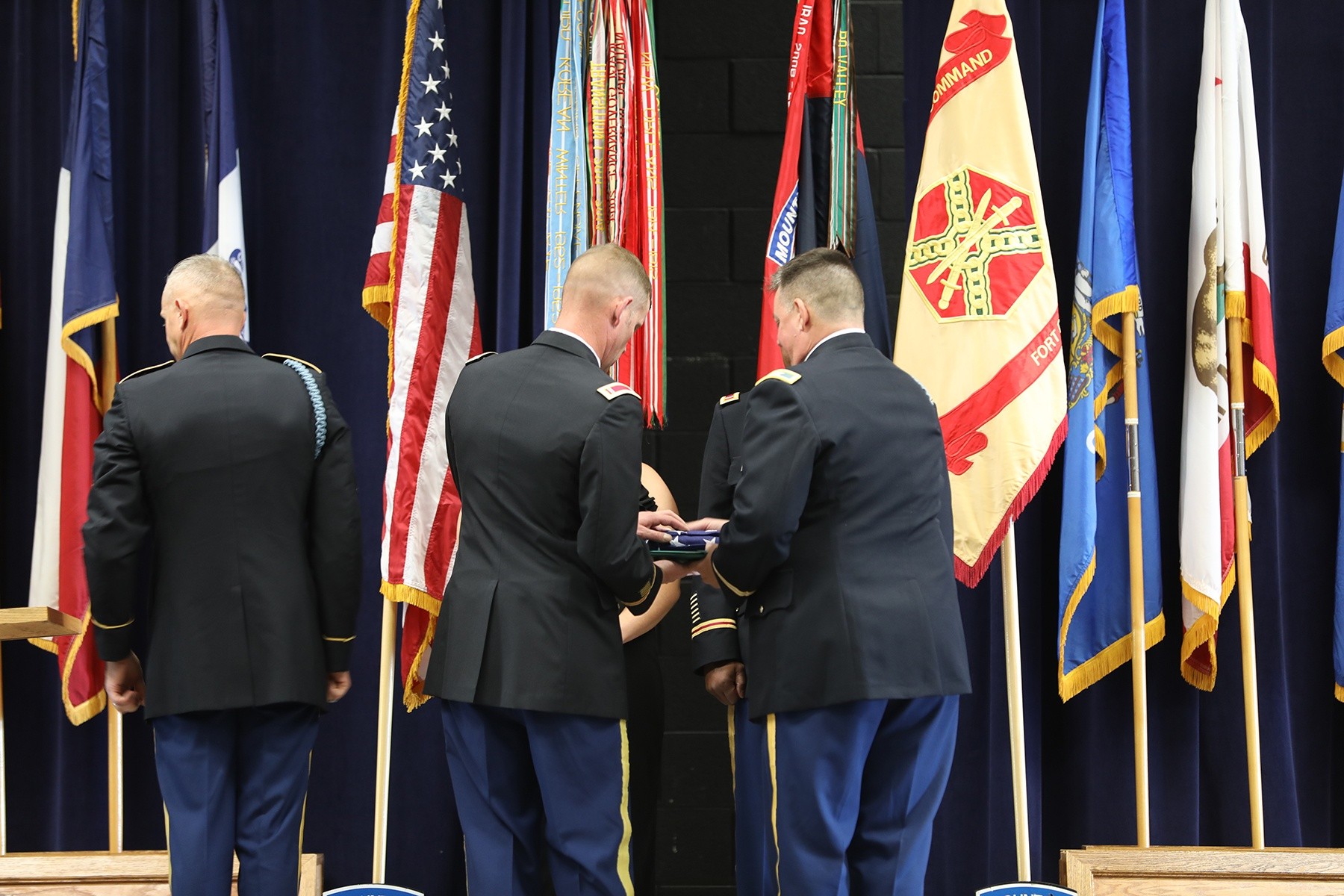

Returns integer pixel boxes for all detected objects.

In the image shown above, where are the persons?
[686,247,975,896]
[423,242,688,893]
[83,254,366,896]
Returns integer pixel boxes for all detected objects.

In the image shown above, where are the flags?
[27,1,121,728]
[1058,0,1165,706]
[196,0,250,345]
[1180,1,1280,694]
[755,0,894,382]
[891,0,1070,589]
[360,1,485,714]
[1321,177,1344,389]
